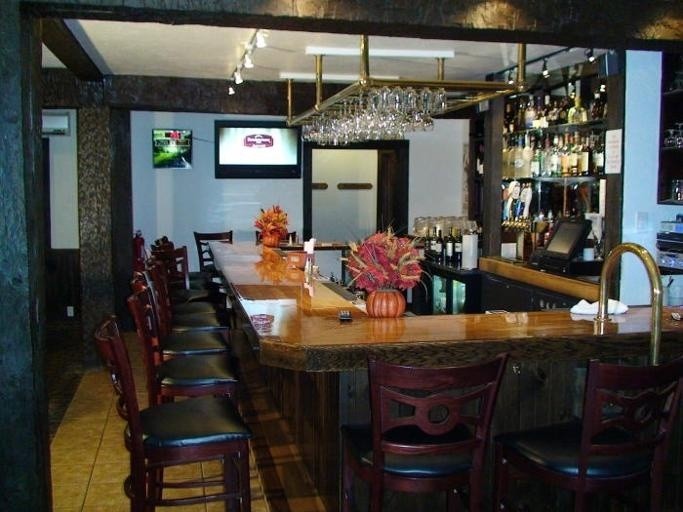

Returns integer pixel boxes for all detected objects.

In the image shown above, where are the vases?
[362,288,406,317]
[262,236,280,247]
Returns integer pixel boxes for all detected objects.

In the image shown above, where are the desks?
[477,243,609,311]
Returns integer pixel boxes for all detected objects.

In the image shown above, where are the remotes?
[339,309,352,322]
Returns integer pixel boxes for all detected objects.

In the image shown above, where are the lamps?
[232,72,244,85]
[228,86,235,96]
[254,30,269,49]
[242,53,254,70]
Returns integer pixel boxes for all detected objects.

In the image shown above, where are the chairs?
[490,359,682,511]
[169,246,214,304]
[128,278,240,406]
[136,257,231,342]
[189,230,232,304]
[93,316,256,512]
[148,237,218,313]
[337,350,510,510]
[131,272,234,356]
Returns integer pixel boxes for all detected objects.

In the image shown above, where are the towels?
[569,298,628,315]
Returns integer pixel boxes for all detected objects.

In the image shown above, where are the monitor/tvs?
[542,218,592,260]
[213,119,302,179]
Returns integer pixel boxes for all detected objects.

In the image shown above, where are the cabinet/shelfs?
[471,26,626,300]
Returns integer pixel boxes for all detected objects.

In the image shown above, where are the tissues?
[288,238,317,270]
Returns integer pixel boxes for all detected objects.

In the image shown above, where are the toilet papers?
[461,234,480,269]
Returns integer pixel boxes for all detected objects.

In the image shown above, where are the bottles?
[572,135,580,177]
[591,90,603,120]
[552,135,561,177]
[515,135,523,179]
[581,136,586,147]
[508,137,516,179]
[455,229,463,262]
[567,96,587,125]
[531,146,542,178]
[502,137,508,179]
[522,134,534,178]
[430,227,437,256]
[540,132,551,178]
[503,103,513,133]
[424,229,431,253]
[559,135,564,150]
[444,227,456,261]
[435,229,444,262]
[561,133,572,178]
[592,136,605,175]
[581,137,594,177]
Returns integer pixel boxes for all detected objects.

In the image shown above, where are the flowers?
[249,203,291,237]
[341,225,435,296]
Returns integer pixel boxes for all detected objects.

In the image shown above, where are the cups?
[674,130,683,147]
[301,86,448,146]
[675,122,683,147]
[663,129,677,147]
[674,71,683,89]
[414,216,477,237]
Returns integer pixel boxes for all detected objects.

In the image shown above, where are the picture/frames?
[153,128,191,169]
